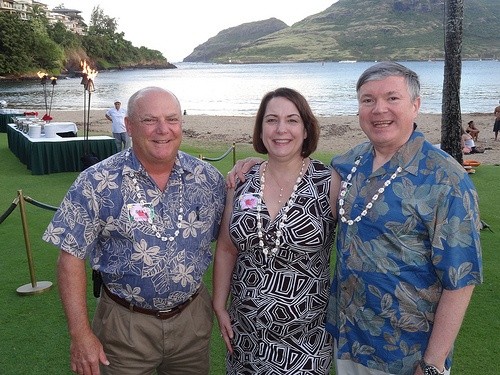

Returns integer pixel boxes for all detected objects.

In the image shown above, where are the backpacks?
[471,145,485,153]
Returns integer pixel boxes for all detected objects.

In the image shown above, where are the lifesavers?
[463,160,481,167]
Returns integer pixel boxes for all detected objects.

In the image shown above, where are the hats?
[114,99,122,103]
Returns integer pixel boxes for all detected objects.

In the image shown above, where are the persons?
[461,127,475,148]
[227,63,483,375]
[212,88,343,375]
[493,100,500,141]
[42,87,227,375]
[466,121,480,142]
[105,99,128,153]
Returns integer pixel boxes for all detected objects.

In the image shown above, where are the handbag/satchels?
[462,145,472,154]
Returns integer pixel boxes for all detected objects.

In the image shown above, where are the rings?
[221,334,222,337]
[228,172,230,174]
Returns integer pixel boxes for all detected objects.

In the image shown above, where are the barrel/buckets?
[29,125,41,138]
[44,124,56,138]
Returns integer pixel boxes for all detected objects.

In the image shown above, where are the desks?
[7,122,118,175]
[0,108,36,132]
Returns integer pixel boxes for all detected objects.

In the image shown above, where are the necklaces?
[339,156,402,225]
[256,157,305,254]
[130,173,183,241]
[266,159,302,205]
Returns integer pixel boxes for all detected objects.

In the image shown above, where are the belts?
[101,281,200,320]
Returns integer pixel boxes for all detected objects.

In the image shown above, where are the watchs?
[418,357,447,375]
